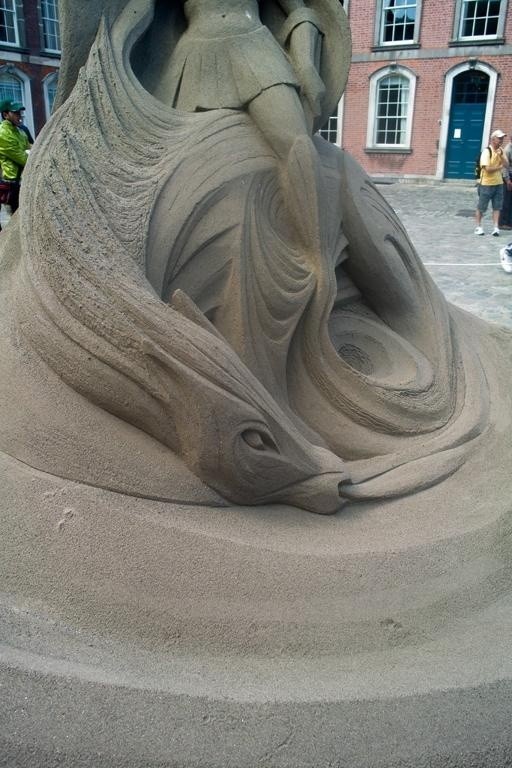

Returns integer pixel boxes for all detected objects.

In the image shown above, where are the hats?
[491,130,507,140]
[0,99,25,112]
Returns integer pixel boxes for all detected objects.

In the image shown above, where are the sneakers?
[474,227,485,235]
[492,228,500,236]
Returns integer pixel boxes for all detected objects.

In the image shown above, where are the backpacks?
[474,147,503,178]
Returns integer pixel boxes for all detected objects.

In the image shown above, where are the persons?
[474,130,512,236]
[0,97,34,232]
[151,0,326,164]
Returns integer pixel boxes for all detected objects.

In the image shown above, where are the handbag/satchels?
[0,179,20,204]
